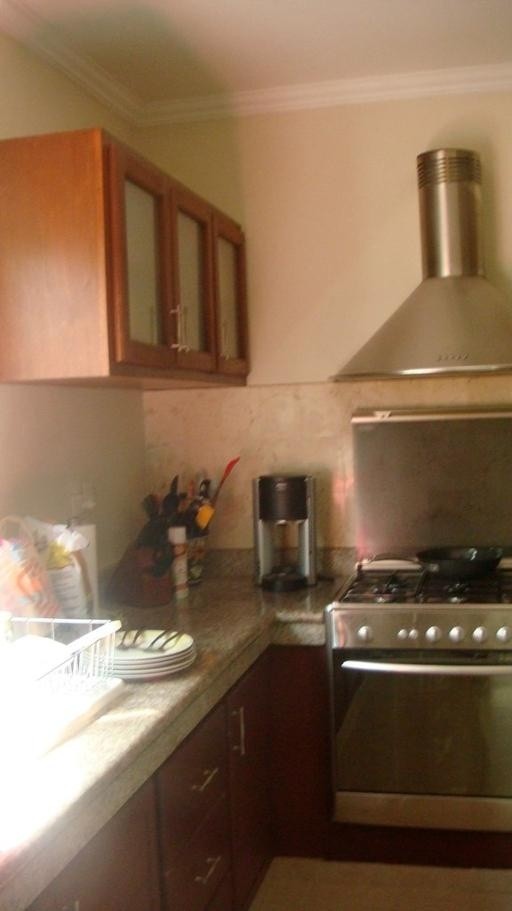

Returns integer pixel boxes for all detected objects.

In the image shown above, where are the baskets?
[0,616,121,767]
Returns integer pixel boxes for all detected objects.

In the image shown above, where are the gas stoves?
[338,565,512,604]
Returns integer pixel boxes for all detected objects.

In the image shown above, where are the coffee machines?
[252,474,317,593]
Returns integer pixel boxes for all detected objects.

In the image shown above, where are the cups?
[187,534,209,588]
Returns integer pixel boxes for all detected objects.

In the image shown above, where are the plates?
[86,629,197,679]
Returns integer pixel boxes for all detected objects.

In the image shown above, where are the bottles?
[167,526,190,601]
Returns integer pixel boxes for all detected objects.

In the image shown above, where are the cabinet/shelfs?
[0,128,216,392]
[29,775,162,911]
[214,205,251,389]
[228,647,279,911]
[157,701,235,911]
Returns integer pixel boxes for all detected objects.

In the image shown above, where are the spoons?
[115,624,185,654]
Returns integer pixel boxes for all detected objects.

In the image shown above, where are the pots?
[416,545,504,583]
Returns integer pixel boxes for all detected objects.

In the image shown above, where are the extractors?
[330,148,512,382]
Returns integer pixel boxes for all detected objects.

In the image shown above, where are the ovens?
[325,604,512,800]
